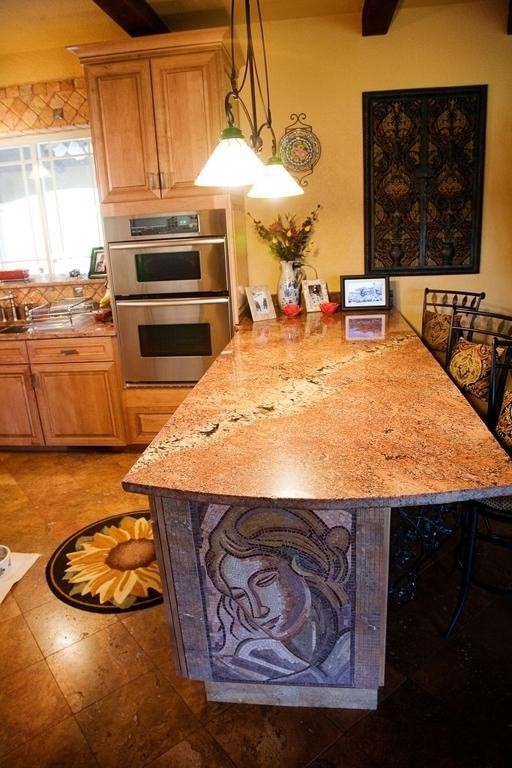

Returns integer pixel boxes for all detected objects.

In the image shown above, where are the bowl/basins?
[0,545,12,579]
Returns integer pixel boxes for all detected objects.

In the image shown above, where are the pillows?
[495,390,512,448]
[423,310,461,350]
[450,337,507,402]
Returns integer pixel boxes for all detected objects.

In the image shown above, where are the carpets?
[46,509,163,613]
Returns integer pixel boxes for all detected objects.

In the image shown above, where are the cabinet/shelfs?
[124,388,194,445]
[83,49,237,204]
[0,337,127,447]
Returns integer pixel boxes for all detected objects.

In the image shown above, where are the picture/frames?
[340,275,392,311]
[244,285,277,322]
[340,312,390,344]
[89,247,109,278]
[302,281,329,313]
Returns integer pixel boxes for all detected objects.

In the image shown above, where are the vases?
[277,261,300,312]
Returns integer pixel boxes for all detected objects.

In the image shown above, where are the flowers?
[247,205,319,280]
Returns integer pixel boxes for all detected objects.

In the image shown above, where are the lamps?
[194,0,305,201]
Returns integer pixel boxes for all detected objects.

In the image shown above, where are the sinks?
[0,325,28,334]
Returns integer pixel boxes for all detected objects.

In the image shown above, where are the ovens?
[103,210,234,390]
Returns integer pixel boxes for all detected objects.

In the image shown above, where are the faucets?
[0,290,19,321]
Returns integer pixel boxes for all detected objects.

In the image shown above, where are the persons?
[312,285,319,301]
[96,255,106,272]
[255,292,267,310]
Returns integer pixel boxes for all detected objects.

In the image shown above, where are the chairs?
[442,338,512,638]
[422,287,486,353]
[447,309,512,399]
